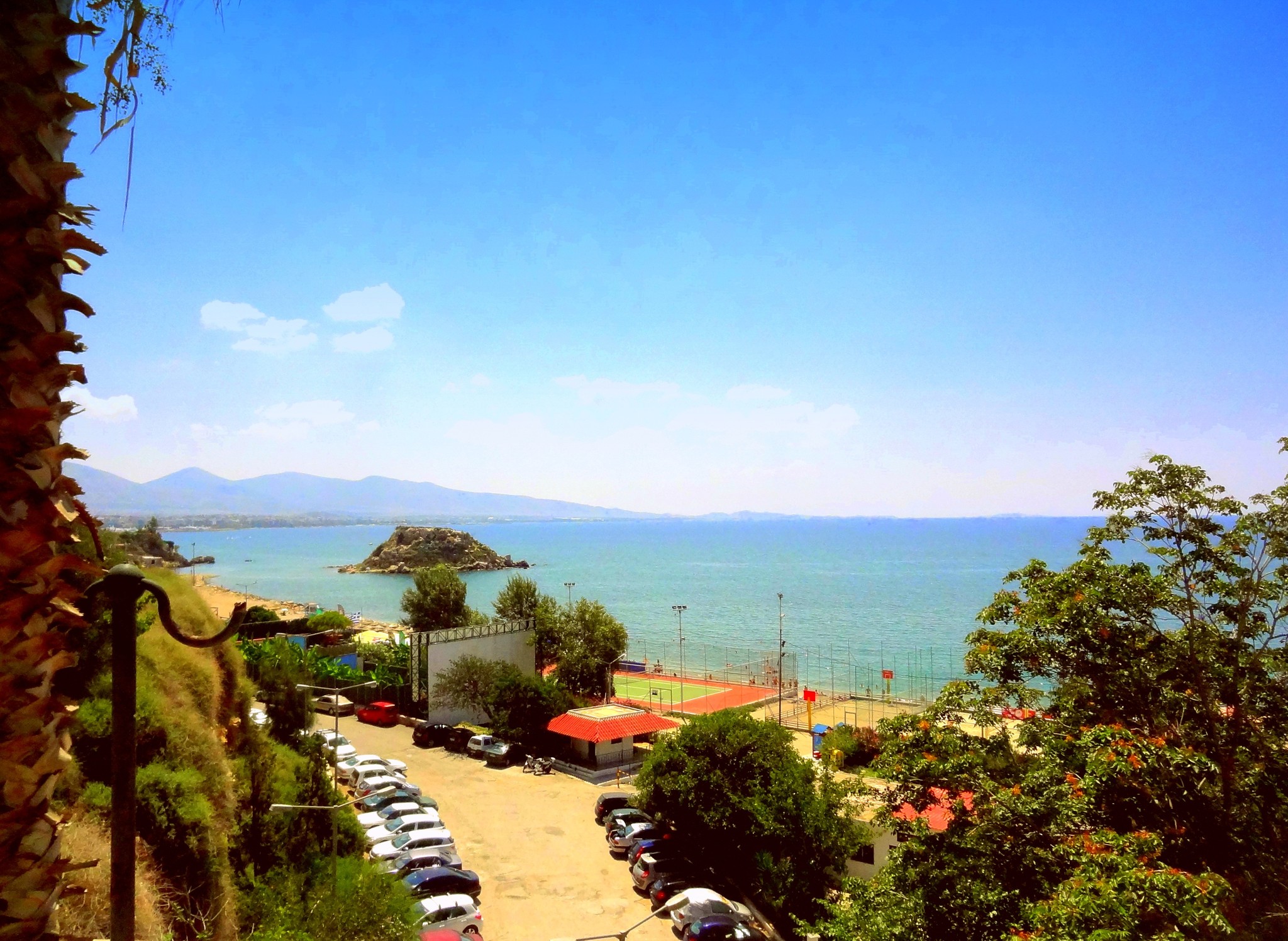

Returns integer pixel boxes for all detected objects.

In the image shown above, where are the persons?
[727,656,828,697]
[709,674,712,679]
[674,673,676,676]
[861,684,878,697]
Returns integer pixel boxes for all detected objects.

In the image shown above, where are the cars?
[608,822,731,908]
[606,808,653,834]
[413,721,522,766]
[310,694,397,726]
[670,888,769,941]
[594,792,638,824]
[336,755,482,901]
[248,708,271,727]
[418,929,484,941]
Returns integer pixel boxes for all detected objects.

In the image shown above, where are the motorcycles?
[523,754,556,775]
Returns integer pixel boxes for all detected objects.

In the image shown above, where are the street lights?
[672,606,687,713]
[777,592,783,727]
[269,785,396,895]
[296,680,377,792]
[236,582,257,604]
[191,542,196,584]
[564,583,576,603]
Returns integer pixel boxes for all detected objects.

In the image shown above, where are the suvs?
[311,728,357,764]
[382,892,484,937]
